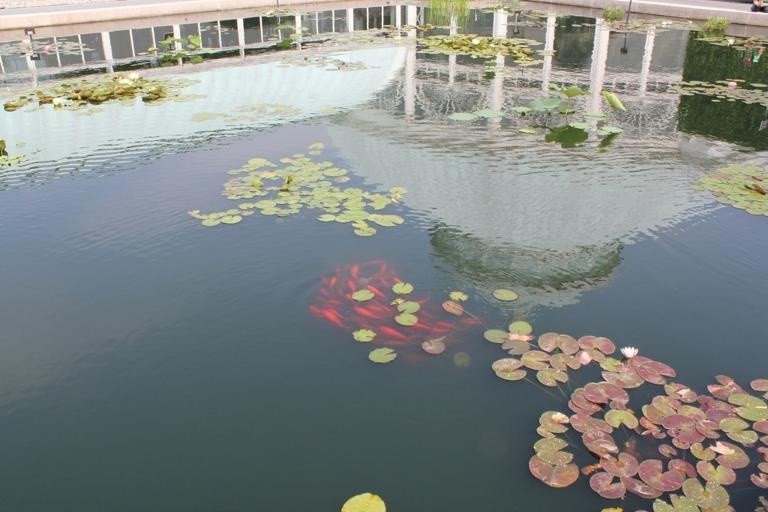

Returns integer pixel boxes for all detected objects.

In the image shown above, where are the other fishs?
[457,316,485,326]
